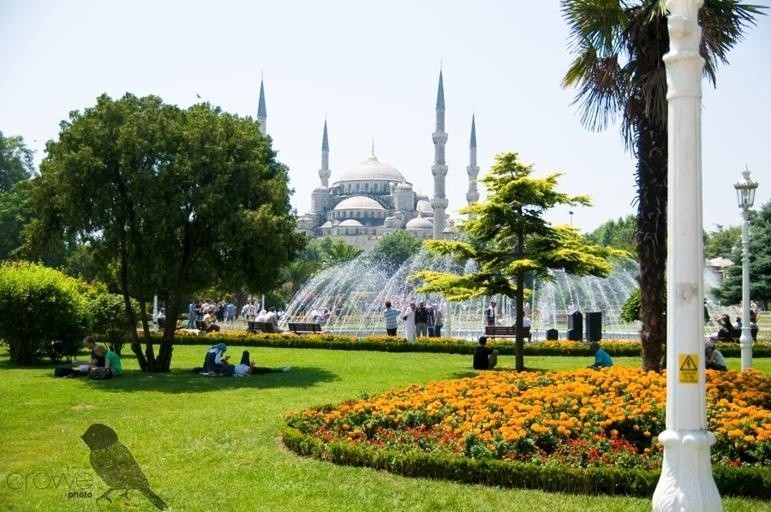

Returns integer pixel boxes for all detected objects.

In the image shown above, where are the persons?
[512,311,531,342]
[204,342,226,374]
[403,300,443,342]
[587,343,613,371]
[94,345,121,376]
[705,315,757,371]
[473,337,498,369]
[486,300,497,326]
[82,336,105,367]
[226,351,290,375]
[383,302,401,336]
[158,298,330,334]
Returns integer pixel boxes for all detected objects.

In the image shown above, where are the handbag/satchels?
[54,367,74,376]
[90,366,112,379]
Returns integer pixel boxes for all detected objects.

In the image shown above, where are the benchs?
[483,326,532,343]
[712,328,758,344]
[158,318,166,331]
[248,321,273,332]
[196,321,212,334]
[288,322,322,336]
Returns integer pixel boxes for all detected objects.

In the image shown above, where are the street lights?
[732,162,759,371]
